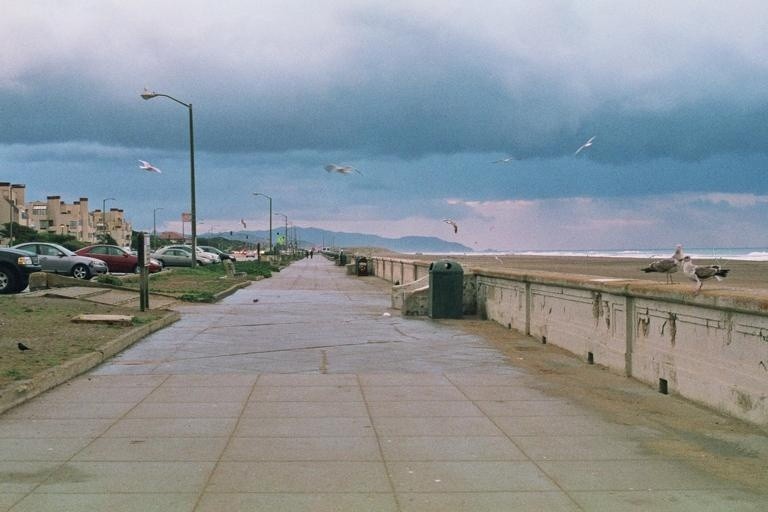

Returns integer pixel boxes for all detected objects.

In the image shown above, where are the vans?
[321,246,331,254]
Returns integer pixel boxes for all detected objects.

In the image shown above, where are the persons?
[310,251,313,258]
[219,254,235,276]
[305,250,309,259]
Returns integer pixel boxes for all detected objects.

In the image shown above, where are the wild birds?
[679,255,730,298]
[240,218,249,230]
[137,159,161,174]
[442,219,458,234]
[489,156,517,166]
[18,343,31,352]
[324,164,353,176]
[574,135,595,154]
[640,245,684,285]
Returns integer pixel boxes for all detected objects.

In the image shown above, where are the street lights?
[103,198,117,240]
[141,89,197,268]
[253,192,273,264]
[152,207,163,250]
[8,185,25,248]
[274,212,298,262]
[59,224,64,234]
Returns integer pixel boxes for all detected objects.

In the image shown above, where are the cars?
[246,250,255,258]
[0,247,41,294]
[11,242,108,281]
[72,244,237,275]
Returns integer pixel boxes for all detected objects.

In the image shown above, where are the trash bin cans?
[355,257,368,276]
[335,254,339,259]
[429,261,463,319]
[339,255,346,265]
